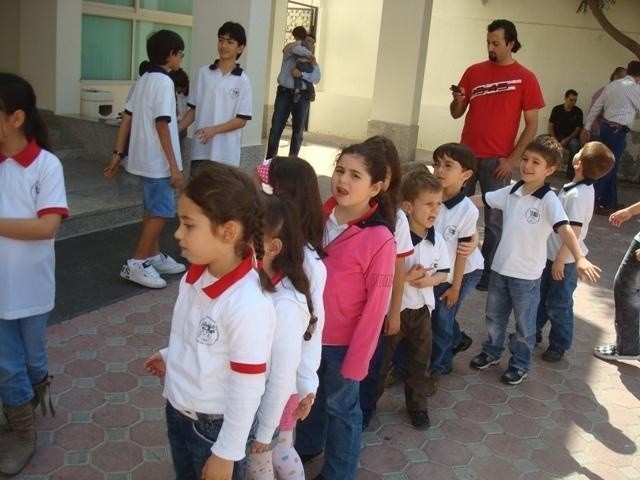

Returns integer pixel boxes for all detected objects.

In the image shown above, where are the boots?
[1,375,50,477]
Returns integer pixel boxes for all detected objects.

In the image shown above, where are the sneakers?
[542,348,563,362]
[120,257,168,289]
[146,252,187,275]
[377,331,473,400]
[592,344,639,360]
[410,409,431,430]
[469,350,500,370]
[502,366,528,385]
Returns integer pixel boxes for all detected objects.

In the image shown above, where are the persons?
[285,26,315,103]
[262,34,320,196]
[294,135,485,479]
[236,156,328,479]
[178,22,251,178]
[451,19,546,290]
[593,200,640,362]
[466,133,602,384]
[121,60,149,174]
[583,61,640,215]
[144,158,279,478]
[1,71,68,477]
[587,68,627,201]
[168,68,195,122]
[548,89,581,182]
[519,140,615,362]
[104,30,184,288]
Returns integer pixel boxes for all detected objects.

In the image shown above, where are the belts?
[284,87,308,94]
[602,119,622,129]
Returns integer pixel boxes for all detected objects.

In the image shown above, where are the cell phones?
[452,84,463,96]
